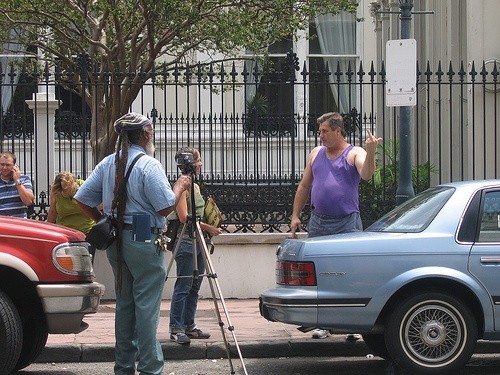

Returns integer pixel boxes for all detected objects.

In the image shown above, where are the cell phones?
[14,163,18,171]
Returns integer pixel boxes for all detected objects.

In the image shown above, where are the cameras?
[175,153,196,167]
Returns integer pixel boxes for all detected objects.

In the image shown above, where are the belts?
[122,224,160,235]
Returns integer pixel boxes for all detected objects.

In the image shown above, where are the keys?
[153,230,172,257]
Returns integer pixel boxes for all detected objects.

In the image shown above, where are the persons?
[167,145,223,346]
[72,112,195,375]
[46,170,103,266]
[0,151,34,220]
[290,111,384,341]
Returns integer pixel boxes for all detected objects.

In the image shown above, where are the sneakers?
[346,333,360,341]
[312,328,335,339]
[169,332,191,344]
[185,329,210,339]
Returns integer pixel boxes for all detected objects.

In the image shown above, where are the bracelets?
[15,182,22,187]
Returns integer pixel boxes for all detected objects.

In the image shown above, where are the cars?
[258,178,500,375]
[0,214,105,375]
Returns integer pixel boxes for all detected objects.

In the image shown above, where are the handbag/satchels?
[164,219,179,251]
[85,215,120,251]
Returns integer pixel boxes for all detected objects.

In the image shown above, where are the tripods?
[163,169,249,375]
